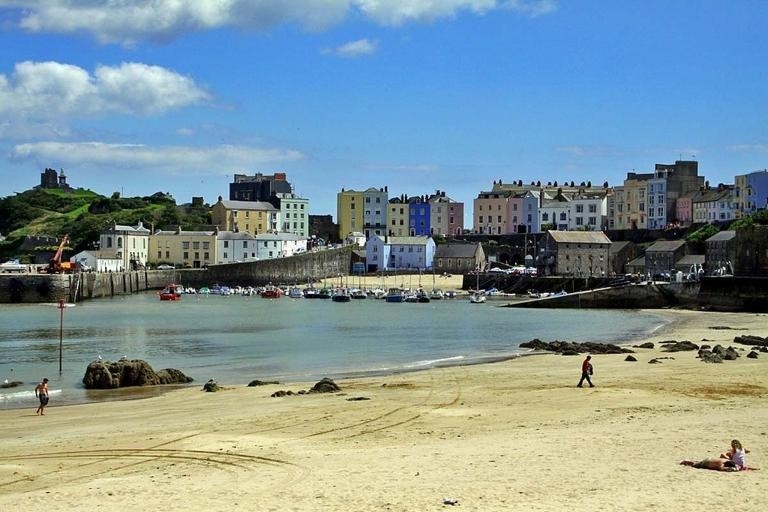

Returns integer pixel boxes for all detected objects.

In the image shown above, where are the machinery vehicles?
[45,234,76,276]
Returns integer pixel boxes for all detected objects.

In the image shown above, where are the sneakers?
[577,385,595,387]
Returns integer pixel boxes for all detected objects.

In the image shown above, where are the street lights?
[57,298,63,372]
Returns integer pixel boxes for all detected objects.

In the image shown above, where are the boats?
[526,288,567,299]
[199,276,300,298]
[159,284,196,301]
[468,287,516,303]
[372,287,456,303]
[303,287,368,301]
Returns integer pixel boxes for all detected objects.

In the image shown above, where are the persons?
[679,455,740,472]
[718,440,750,466]
[575,356,595,387]
[34,377,49,416]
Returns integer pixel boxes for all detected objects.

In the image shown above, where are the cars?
[157,264,175,269]
